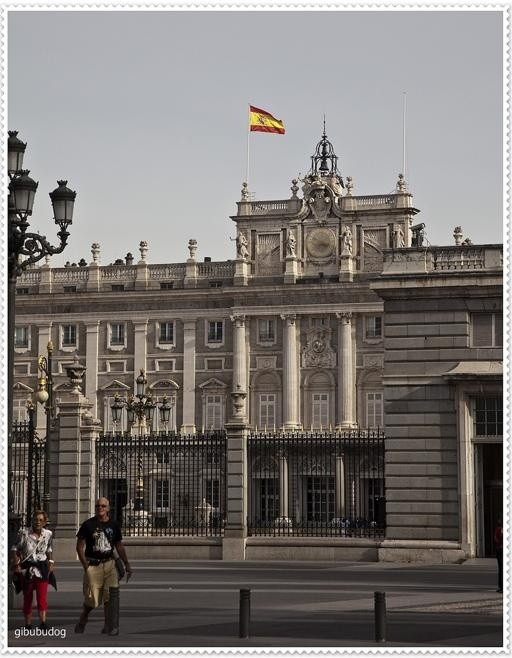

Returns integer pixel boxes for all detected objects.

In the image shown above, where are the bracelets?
[48,558,55,564]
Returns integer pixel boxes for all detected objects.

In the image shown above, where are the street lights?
[34,341,54,530]
[110,369,170,509]
[8,131,74,607]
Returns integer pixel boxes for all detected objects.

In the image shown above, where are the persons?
[75,494,132,632]
[10,509,55,631]
[337,516,377,537]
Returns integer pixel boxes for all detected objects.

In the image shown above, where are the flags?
[250,106,286,137]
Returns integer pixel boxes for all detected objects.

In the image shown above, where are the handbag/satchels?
[115,557,126,576]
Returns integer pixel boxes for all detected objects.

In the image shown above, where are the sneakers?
[74,622,86,634]
[100,624,112,634]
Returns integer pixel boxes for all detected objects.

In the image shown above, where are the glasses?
[95,504,106,508]
